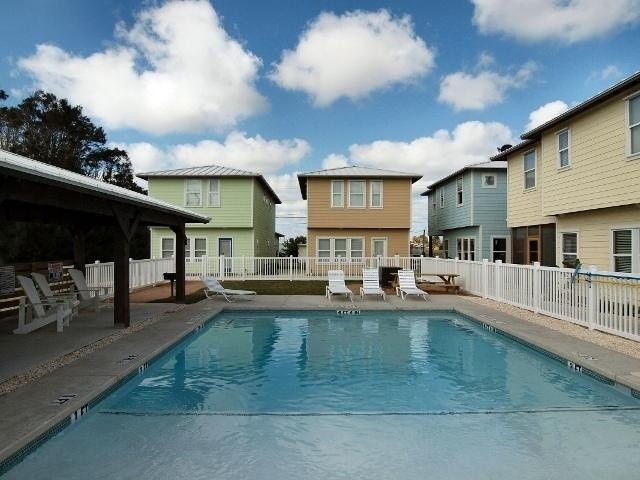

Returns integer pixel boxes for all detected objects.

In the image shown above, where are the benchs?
[416,273,461,293]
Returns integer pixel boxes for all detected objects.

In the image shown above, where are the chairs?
[325,266,427,302]
[3,261,133,337]
[200,274,257,304]
